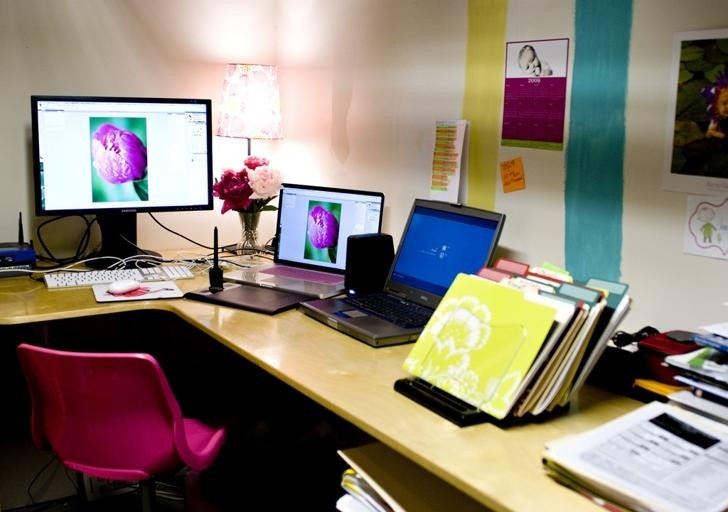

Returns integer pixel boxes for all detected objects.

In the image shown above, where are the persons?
[675,88,728,145]
[516,44,554,76]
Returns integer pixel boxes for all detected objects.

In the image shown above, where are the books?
[336,444,491,512]
[404,260,633,422]
[542,322,725,511]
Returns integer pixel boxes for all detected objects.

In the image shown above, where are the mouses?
[108,279,140,294]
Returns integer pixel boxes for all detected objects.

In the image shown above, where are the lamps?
[215,62,284,254]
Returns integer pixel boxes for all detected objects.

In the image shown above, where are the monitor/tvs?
[30,94,214,270]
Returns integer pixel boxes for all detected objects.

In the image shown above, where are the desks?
[0,236,728,512]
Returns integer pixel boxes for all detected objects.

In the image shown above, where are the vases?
[238,211,262,255]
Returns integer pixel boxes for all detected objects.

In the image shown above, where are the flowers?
[213,156,283,214]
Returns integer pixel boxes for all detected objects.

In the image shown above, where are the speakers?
[343,233,394,296]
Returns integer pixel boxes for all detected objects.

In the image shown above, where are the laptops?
[296,198,505,347]
[223,183,385,300]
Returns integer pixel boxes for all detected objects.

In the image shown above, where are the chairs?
[18,343,226,512]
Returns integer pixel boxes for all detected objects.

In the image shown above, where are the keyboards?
[44,265,194,289]
